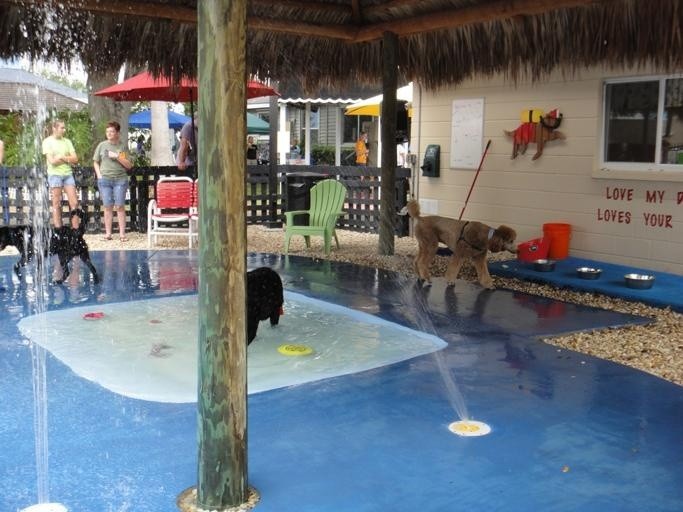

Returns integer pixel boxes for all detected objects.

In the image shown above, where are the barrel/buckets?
[543,223,571,259]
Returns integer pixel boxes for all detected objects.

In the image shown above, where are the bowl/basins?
[534,258,556,271]
[623,273,654,289]
[576,267,601,279]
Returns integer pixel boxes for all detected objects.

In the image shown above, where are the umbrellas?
[127,110,192,129]
[94,71,282,179]
[247,112,270,134]
[344,86,412,118]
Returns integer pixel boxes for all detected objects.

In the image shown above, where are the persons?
[356,132,369,180]
[290,138,301,158]
[137,135,146,154]
[53,254,79,303]
[177,111,198,171]
[92,122,134,242]
[96,250,128,302]
[41,120,79,229]
[247,136,259,166]
[0,140,4,164]
[397,144,405,168]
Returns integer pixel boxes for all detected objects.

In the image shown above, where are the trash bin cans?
[282,172,324,226]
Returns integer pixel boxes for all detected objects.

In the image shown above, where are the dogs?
[0,208,99,285]
[397,199,519,290]
[247,267,284,345]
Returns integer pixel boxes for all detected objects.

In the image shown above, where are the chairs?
[280,178,350,258]
[188,178,198,250]
[146,176,193,253]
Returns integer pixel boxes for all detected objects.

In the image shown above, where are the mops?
[436,139,492,257]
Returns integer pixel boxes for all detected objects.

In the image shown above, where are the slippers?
[99,235,130,242]
